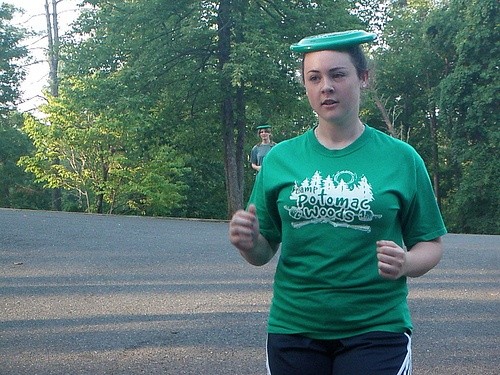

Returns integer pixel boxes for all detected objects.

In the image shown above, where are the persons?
[229,44,447,375]
[250,128,278,172]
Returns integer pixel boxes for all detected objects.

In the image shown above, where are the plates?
[257,125,272,128]
[290,30,377,52]
